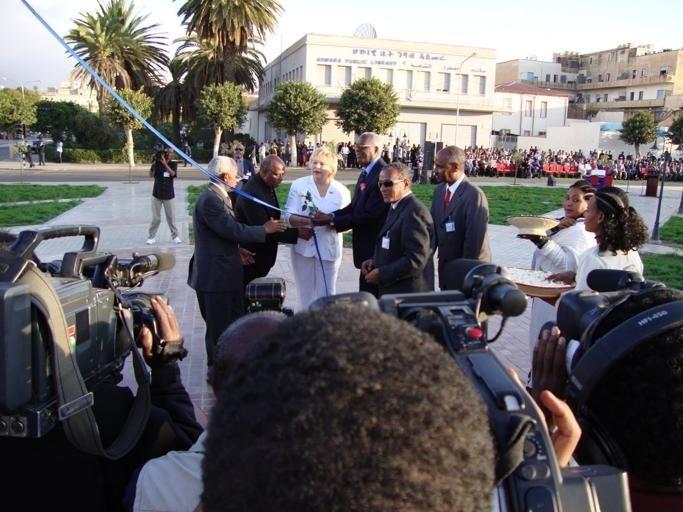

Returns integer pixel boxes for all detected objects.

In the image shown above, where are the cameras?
[247,277,294,318]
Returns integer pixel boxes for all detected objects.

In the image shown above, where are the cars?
[25,133,44,153]
[0,128,52,140]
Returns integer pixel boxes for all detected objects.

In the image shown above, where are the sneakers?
[172,237,182,243]
[146,238,154,244]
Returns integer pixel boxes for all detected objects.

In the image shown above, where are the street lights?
[648,130,675,241]
[2,77,43,98]
[454,51,480,144]
[387,126,408,163]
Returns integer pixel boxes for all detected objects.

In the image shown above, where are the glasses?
[235,148,243,151]
[352,143,372,148]
[378,180,404,187]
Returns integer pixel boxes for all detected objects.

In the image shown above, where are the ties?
[444,190,452,208]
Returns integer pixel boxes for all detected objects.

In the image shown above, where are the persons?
[144,144,182,244]
[150,141,164,163]
[0,294,203,511]
[234,155,313,285]
[528,179,597,366]
[221,138,425,182]
[192,302,584,510]
[197,140,204,159]
[118,308,294,512]
[429,145,488,291]
[529,282,682,510]
[542,185,649,291]
[188,157,287,386]
[465,146,682,182]
[362,161,435,298]
[284,146,351,310]
[311,131,388,299]
[26,144,34,168]
[181,141,191,166]
[55,138,64,163]
[37,137,45,166]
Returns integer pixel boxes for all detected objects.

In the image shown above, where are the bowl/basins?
[504,214,559,238]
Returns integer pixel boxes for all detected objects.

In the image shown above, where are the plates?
[502,266,577,299]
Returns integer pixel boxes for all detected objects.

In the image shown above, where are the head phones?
[557,301,682,475]
